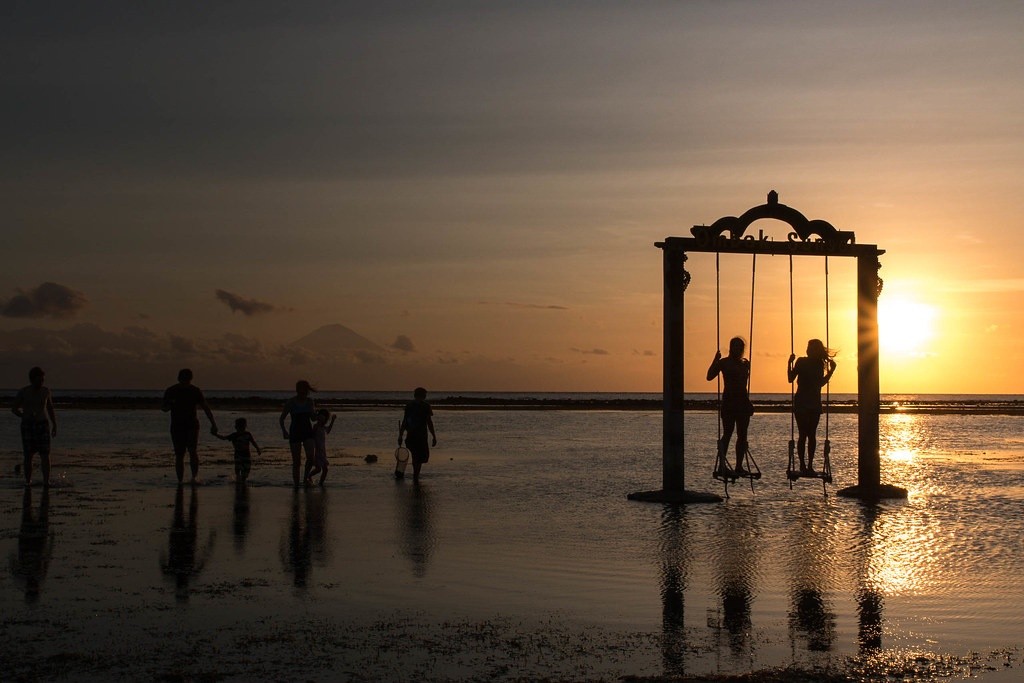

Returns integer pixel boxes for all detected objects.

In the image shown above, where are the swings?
[787,255,834,483]
[714,254,762,482]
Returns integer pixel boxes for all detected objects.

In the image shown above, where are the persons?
[707,337,752,476]
[160,368,262,485]
[398,387,437,481]
[279,380,337,488]
[788,339,836,474]
[12,366,58,492]
